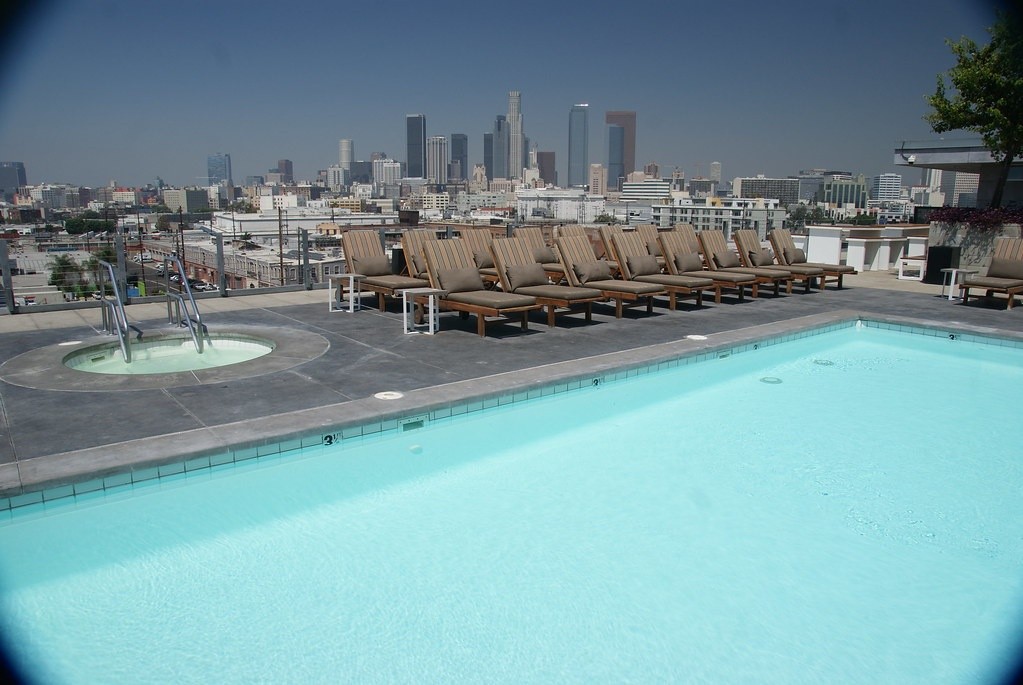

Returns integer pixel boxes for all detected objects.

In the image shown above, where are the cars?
[156,262,232,293]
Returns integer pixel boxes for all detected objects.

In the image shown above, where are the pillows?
[411,254,427,274]
[472,250,494,270]
[713,250,742,268]
[986,255,1023,279]
[532,247,558,263]
[352,254,393,276]
[505,262,549,291]
[646,241,663,256]
[674,252,704,274]
[626,254,661,278]
[437,265,485,298]
[748,248,774,268]
[573,260,614,284]
[784,247,807,266]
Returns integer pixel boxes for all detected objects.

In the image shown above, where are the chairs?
[959,238,1023,310]
[336,220,858,338]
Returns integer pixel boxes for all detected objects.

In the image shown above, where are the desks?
[326,274,367,313]
[400,288,447,335]
[805,224,930,273]
[940,268,980,300]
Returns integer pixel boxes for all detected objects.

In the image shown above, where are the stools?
[899,254,927,281]
